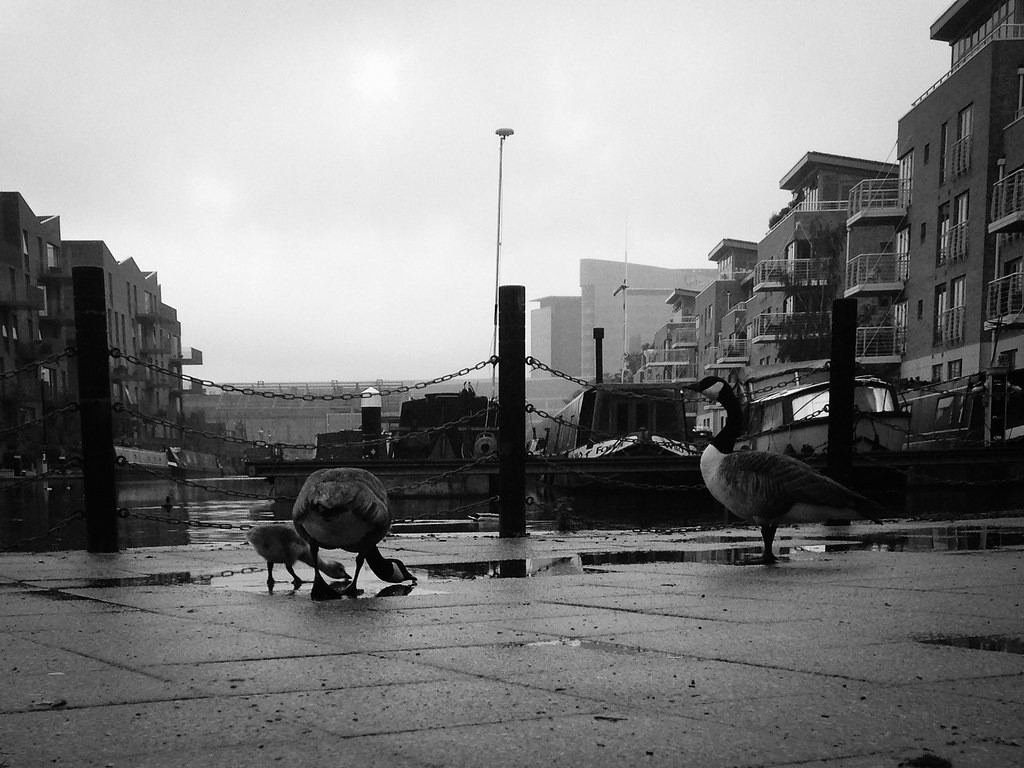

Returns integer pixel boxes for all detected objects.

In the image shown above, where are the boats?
[238,292,1024,514]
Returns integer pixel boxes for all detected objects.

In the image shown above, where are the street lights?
[491,127,516,397]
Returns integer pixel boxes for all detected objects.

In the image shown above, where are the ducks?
[681,374,885,566]
[243,466,418,596]
